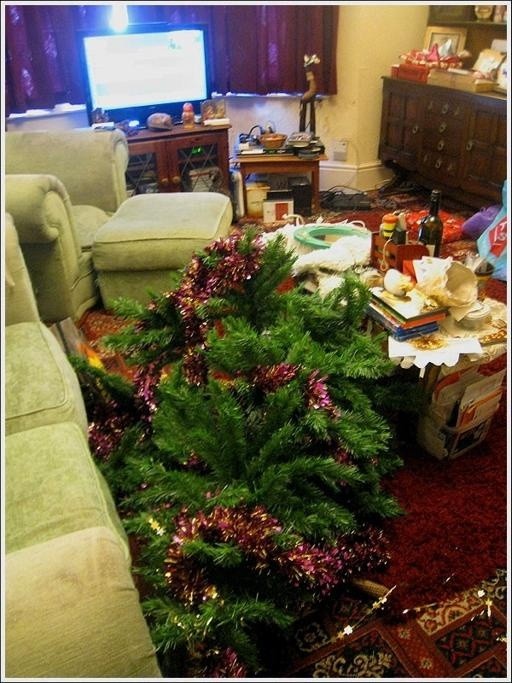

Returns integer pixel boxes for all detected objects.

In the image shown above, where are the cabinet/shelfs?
[382,81,507,200]
[426,5,509,28]
[122,122,233,193]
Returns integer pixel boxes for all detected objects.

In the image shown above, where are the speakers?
[288,176,312,217]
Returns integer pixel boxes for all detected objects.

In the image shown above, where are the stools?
[92,190,233,318]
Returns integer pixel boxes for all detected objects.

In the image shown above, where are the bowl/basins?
[258,133,287,147]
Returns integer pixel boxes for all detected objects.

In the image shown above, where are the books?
[365,282,448,341]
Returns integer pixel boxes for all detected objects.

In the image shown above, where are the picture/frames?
[422,25,468,68]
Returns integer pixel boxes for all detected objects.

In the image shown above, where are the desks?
[233,145,328,216]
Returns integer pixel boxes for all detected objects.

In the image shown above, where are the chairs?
[5,129,129,324]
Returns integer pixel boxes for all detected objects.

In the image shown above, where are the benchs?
[4,319,160,678]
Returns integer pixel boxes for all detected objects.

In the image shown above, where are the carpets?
[333,209,508,620]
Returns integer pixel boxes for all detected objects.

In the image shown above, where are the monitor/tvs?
[76,20,212,130]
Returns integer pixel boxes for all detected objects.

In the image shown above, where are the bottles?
[182,102,195,130]
[417,189,443,258]
